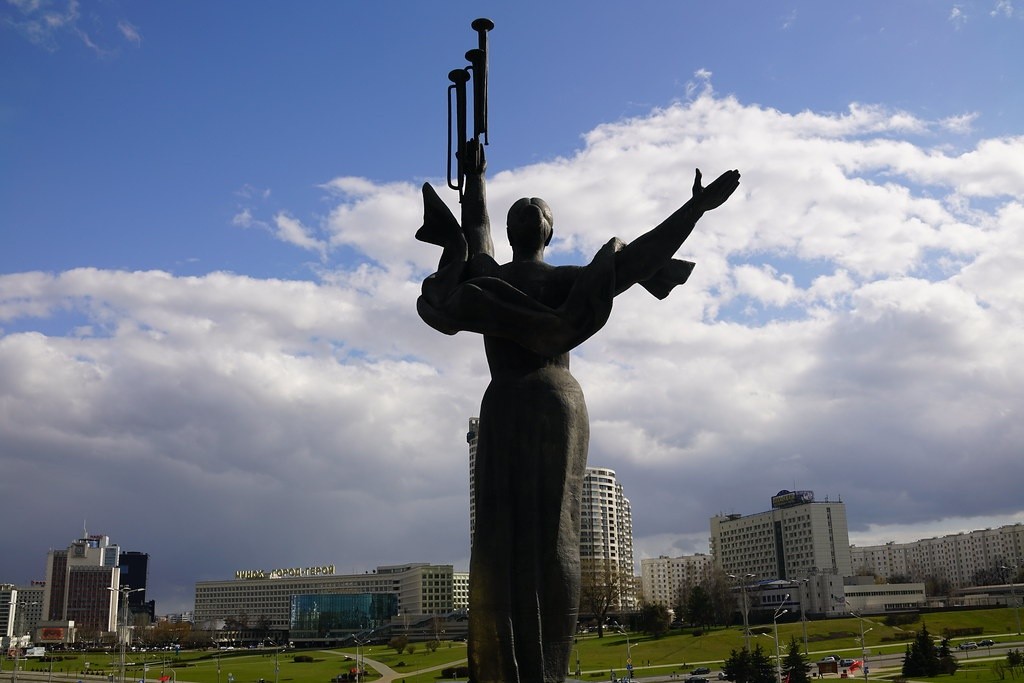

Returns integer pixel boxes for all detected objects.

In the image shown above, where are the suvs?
[961,642,978,650]
[977,640,994,647]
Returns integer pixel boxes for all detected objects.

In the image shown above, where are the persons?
[415,165,741,682]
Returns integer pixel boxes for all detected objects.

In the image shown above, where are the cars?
[690,666,711,676]
[820,654,855,667]
[685,676,710,683]
[718,672,728,680]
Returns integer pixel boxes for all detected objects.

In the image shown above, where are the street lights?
[614,621,639,683]
[268,637,285,683]
[845,601,872,681]
[1001,566,1021,634]
[7,599,38,682]
[351,629,374,683]
[761,594,789,683]
[728,574,757,653]
[106,586,146,683]
[211,637,228,683]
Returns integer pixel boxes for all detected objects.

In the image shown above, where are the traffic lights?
[627,665,633,670]
[630,669,634,679]
